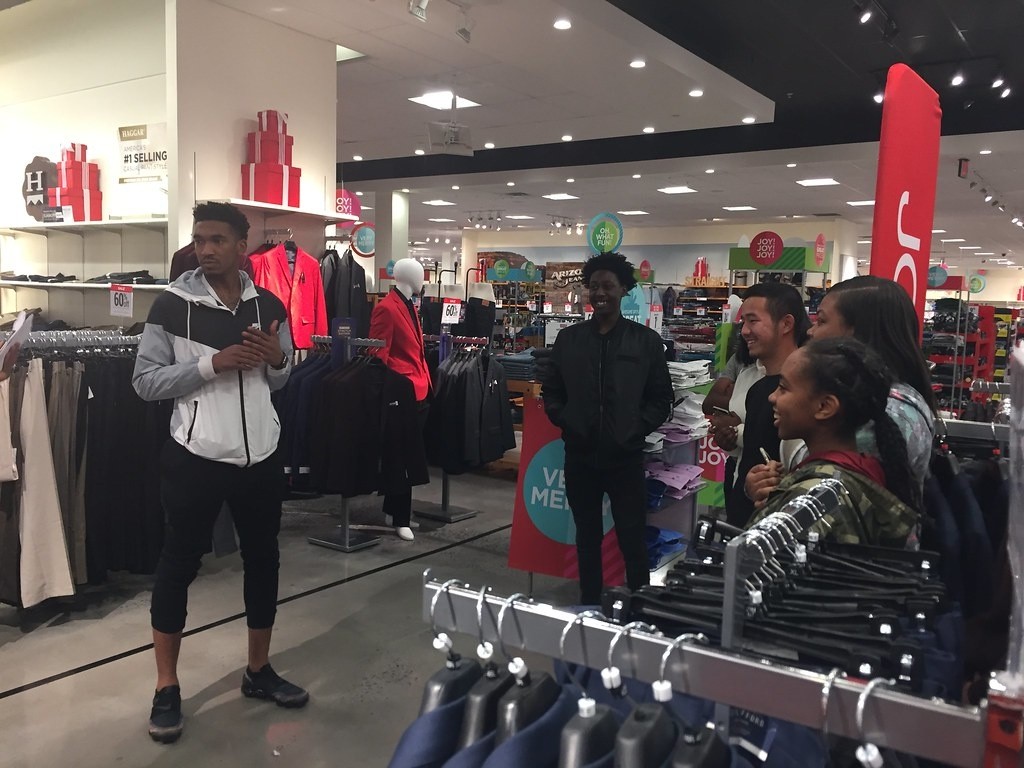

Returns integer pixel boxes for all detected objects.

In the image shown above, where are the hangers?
[969,380,1013,424]
[417,479,988,768]
[931,417,1010,480]
[0,330,144,375]
[265,226,297,263]
[327,235,353,269]
[311,335,386,384]
[422,334,489,372]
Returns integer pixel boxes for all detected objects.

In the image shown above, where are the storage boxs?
[241,110,301,208]
[47,142,102,221]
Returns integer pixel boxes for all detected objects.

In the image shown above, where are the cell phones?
[760,448,771,464]
[712,406,728,415]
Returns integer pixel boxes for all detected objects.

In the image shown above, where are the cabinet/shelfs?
[0,218,168,290]
[644,379,715,585]
[485,379,542,469]
[927,304,1024,408]
[486,260,543,354]
[680,285,750,313]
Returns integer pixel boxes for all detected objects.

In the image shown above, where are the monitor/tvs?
[427,120,474,156]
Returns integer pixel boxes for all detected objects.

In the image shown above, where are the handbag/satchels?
[889,391,993,600]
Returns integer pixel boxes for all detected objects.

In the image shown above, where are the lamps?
[467,210,502,232]
[882,17,901,48]
[970,171,1024,229]
[548,216,585,237]
[408,0,429,23]
[455,6,476,43]
[852,0,874,24]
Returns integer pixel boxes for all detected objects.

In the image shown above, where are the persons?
[132,202,309,744]
[541,250,675,604]
[367,258,434,540]
[702,275,947,552]
[465,283,495,349]
[421,283,468,336]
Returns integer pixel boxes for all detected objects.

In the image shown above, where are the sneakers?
[242,662,311,707]
[147,684,185,743]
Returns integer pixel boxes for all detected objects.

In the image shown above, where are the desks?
[537,315,585,348]
